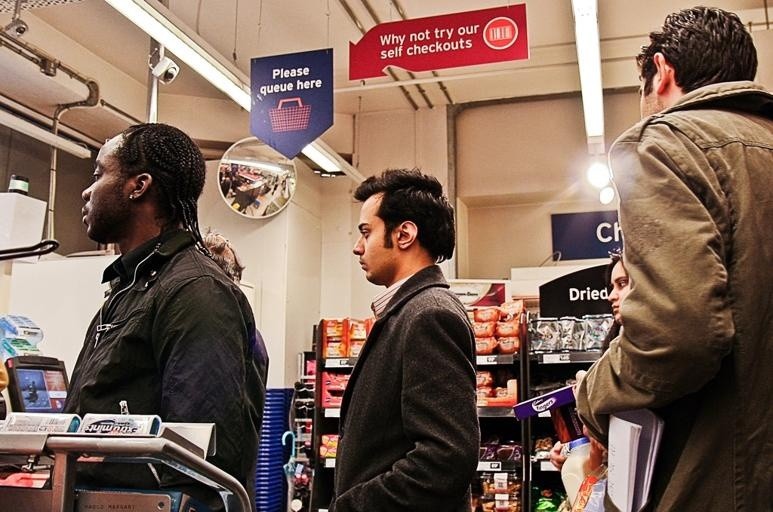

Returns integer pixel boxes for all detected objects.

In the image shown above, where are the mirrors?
[216,137,298,219]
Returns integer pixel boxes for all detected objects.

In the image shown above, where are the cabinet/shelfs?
[288,279,615,512]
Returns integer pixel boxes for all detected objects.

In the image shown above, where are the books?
[605,406,667,511]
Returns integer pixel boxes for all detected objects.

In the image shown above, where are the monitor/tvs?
[4,356,70,413]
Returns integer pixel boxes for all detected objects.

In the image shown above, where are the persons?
[326,168,480,512]
[201,225,270,368]
[551,244,638,472]
[64,121,266,493]
[574,6,772,512]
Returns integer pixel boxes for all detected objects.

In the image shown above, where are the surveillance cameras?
[152,56,179,86]
